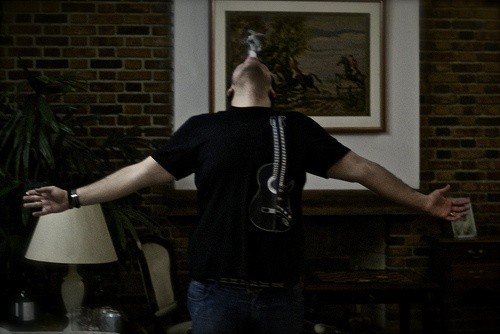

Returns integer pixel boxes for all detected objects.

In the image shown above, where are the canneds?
[99,306,121,334]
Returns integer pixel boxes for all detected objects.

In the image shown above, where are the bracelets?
[66,189,80,209]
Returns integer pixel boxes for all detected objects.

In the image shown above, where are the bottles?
[13,290,41,326]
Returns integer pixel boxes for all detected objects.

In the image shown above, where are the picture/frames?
[208,0,387,135]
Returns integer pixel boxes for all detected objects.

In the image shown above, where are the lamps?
[25,202,118,332]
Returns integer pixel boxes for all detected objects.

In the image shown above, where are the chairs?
[131,235,192,334]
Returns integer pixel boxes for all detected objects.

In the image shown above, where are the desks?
[421,239,500,334]
[302,270,421,334]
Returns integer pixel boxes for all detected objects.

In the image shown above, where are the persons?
[22,59,470,334]
